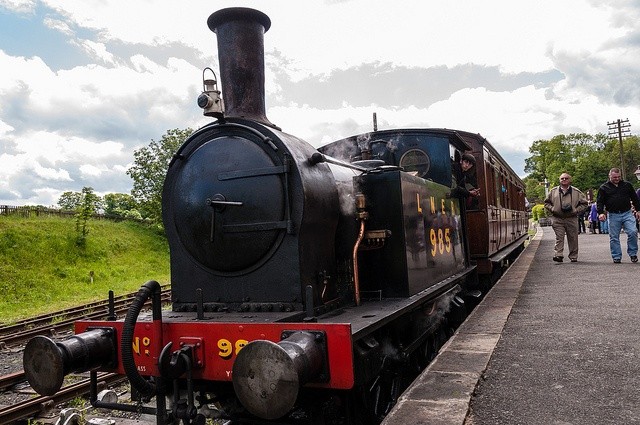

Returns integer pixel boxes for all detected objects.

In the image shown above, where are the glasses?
[561,178,569,180]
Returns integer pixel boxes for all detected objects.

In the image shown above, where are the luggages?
[600,221,608,234]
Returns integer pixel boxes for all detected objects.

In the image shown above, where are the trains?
[23,7,528,425]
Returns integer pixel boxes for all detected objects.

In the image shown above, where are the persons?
[591,202,601,234]
[633,165,640,200]
[544,173,588,262]
[588,200,591,210]
[578,211,585,234]
[591,201,594,206]
[597,168,640,263]
[461,153,480,196]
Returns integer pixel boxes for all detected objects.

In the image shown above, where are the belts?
[612,211,625,214]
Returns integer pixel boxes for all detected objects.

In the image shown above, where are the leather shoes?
[614,260,621,263]
[553,256,563,262]
[571,258,577,262]
[631,256,638,262]
